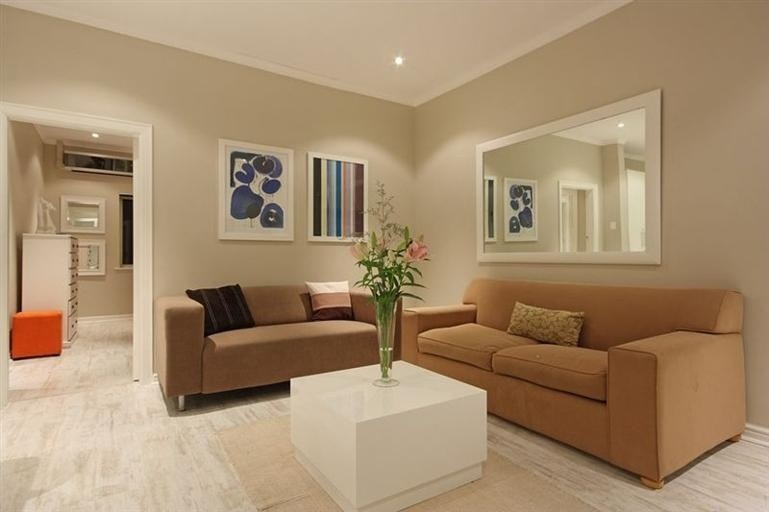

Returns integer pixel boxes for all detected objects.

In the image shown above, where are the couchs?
[401,275,747,490]
[154,281,405,412]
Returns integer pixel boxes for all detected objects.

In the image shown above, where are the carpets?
[216,404,601,512]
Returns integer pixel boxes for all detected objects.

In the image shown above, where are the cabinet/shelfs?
[21,233,80,346]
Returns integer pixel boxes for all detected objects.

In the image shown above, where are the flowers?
[352,180,432,378]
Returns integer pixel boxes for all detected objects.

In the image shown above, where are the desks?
[288,359,487,511]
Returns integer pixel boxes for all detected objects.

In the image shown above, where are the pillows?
[305,281,353,321]
[185,283,255,337]
[505,299,585,348]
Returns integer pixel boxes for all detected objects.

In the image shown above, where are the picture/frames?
[60,194,107,233]
[218,137,294,242]
[306,149,371,245]
[76,238,106,277]
[503,176,539,243]
[483,176,497,243]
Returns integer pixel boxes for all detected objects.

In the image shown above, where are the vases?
[372,299,398,388]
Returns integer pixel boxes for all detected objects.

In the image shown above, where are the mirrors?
[475,88,664,265]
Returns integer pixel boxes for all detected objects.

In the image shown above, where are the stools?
[11,311,63,359]
